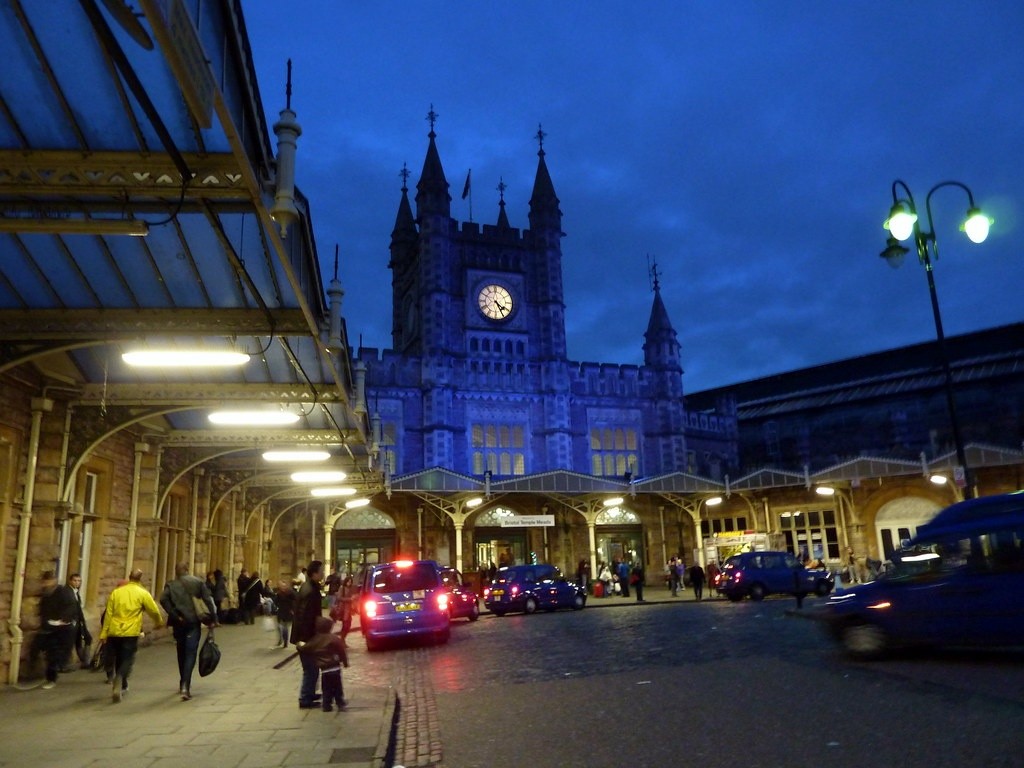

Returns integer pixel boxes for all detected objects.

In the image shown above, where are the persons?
[25,570,93,689]
[689,561,706,601]
[666,555,685,597]
[206,569,230,626]
[579,555,629,597]
[796,546,809,565]
[481,563,497,583]
[632,561,646,601]
[276,560,348,711]
[237,568,276,625]
[499,547,514,567]
[336,576,356,648]
[705,560,721,597]
[161,561,219,701]
[847,545,862,584]
[101,568,162,701]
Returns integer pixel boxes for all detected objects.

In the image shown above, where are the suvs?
[713,551,836,602]
[439,564,480,621]
[357,559,451,652]
[484,563,587,617]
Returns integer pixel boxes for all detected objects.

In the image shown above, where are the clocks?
[476,283,514,319]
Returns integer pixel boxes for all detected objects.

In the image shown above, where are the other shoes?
[182,690,191,700]
[323,706,333,712]
[300,701,321,709]
[122,684,128,690]
[113,691,122,703]
[105,679,112,684]
[313,694,321,701]
[80,663,91,670]
[42,682,56,689]
[278,639,283,646]
[283,644,288,648]
[338,702,348,710]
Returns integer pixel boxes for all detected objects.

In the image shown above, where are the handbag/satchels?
[264,616,275,631]
[89,641,105,673]
[198,629,221,677]
[630,575,639,585]
[192,596,217,626]
[615,583,621,592]
[599,567,612,582]
[329,604,345,621]
[241,592,246,599]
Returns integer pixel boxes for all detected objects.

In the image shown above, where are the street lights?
[877,178,996,561]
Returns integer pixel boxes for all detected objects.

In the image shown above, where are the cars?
[821,491,1024,664]
